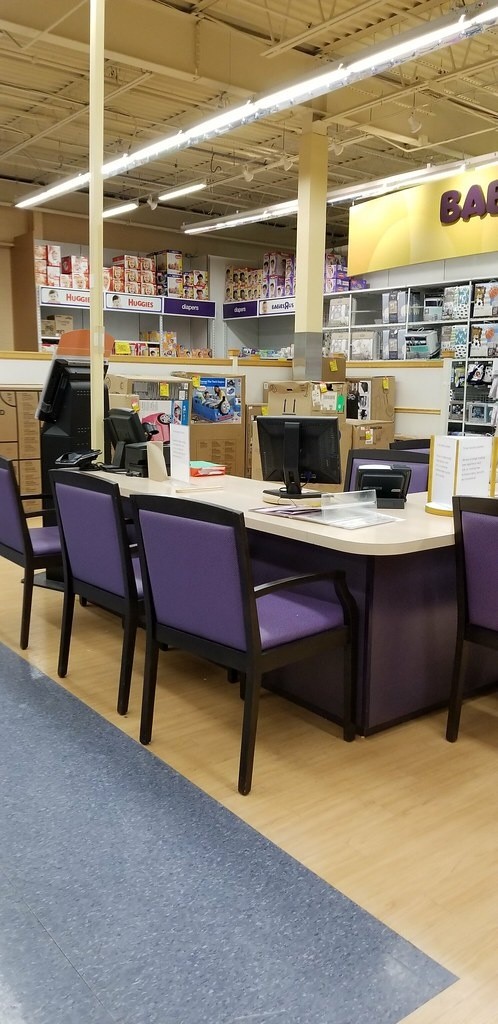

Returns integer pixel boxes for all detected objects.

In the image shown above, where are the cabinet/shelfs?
[25,235,497,440]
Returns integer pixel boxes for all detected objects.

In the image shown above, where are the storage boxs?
[1,373,396,514]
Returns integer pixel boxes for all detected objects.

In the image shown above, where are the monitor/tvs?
[35,357,109,423]
[108,407,146,442]
[256,415,341,484]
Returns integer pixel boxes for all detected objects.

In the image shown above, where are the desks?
[62,461,498,734]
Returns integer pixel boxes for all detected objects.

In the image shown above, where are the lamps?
[11,0,498,210]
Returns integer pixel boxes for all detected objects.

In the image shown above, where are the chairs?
[343,446,430,500]
[129,492,361,803]
[443,494,498,742]
[381,438,432,457]
[46,468,239,715]
[0,456,88,648]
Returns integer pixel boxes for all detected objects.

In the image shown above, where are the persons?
[111,295,121,309]
[261,301,267,313]
[148,335,212,358]
[47,289,61,304]
[224,254,362,301]
[205,391,216,403]
[49,248,206,300]
[213,385,229,403]
